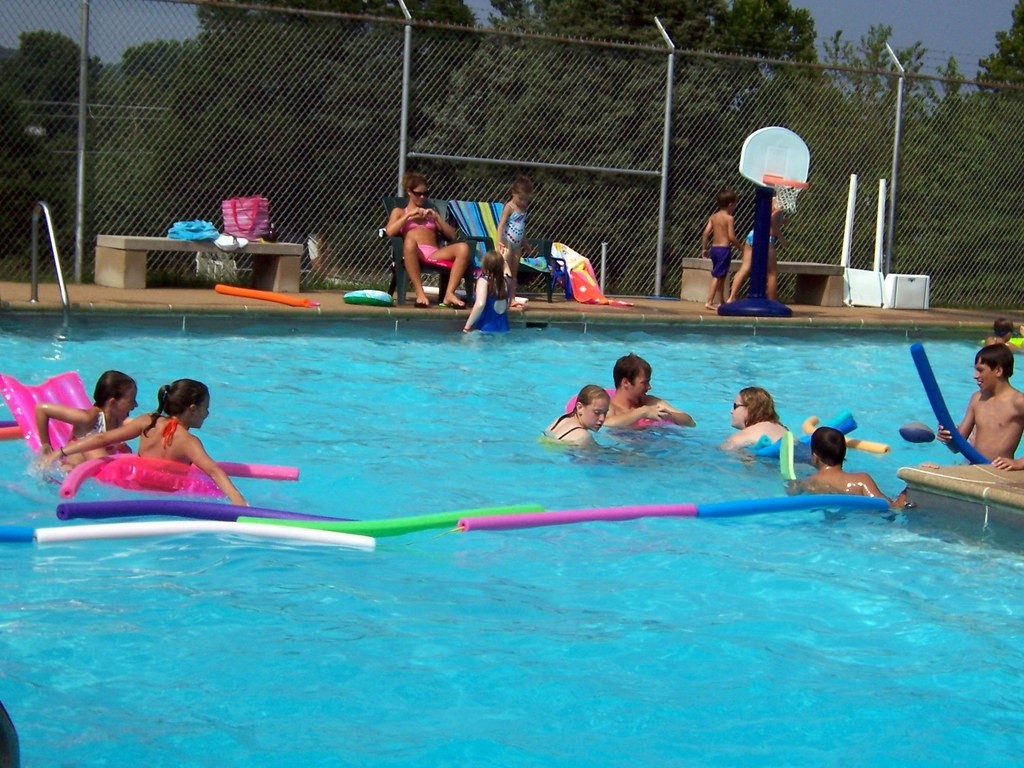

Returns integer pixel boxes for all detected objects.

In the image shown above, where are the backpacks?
[167,219,219,241]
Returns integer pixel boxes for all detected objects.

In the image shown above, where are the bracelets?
[61,447,67,456]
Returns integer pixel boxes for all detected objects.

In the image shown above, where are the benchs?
[680,258,844,306]
[94,236,303,294]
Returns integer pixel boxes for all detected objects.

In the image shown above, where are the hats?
[213,232,249,251]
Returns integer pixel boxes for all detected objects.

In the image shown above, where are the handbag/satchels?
[221,195,268,240]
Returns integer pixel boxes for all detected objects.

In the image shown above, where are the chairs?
[447,200,551,303]
[550,242,597,301]
[379,197,473,305]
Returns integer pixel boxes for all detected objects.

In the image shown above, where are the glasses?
[410,189,429,199]
[733,403,746,411]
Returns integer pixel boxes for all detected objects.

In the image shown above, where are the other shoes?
[447,303,466,309]
[415,303,427,307]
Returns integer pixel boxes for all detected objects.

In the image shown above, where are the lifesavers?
[803,416,891,454]
[779,430,795,475]
[909,340,992,464]
[215,284,310,307]
[0,370,226,498]
[0,492,892,550]
[59,453,302,499]
[982,336,1024,348]
[746,413,858,456]
[566,387,677,429]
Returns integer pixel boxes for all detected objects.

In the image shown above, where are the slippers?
[507,303,526,308]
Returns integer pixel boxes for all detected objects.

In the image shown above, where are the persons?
[386,174,469,310]
[544,383,610,447]
[727,190,788,302]
[986,317,1017,354]
[463,250,511,336]
[497,175,533,307]
[786,427,939,513]
[39,379,247,507]
[600,351,696,430]
[702,190,743,310]
[35,370,138,474]
[720,385,800,452]
[936,343,1024,471]
